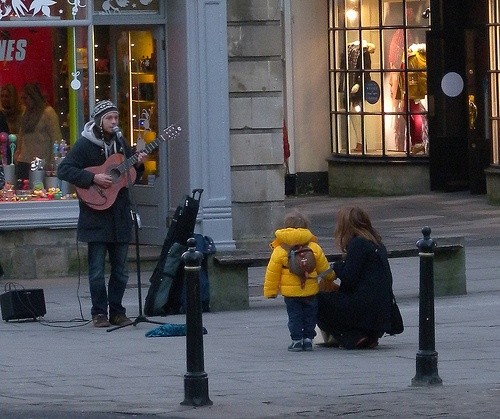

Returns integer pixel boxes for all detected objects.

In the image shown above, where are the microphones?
[112,126,123,142]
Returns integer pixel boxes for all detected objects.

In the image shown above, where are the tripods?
[107,143,169,332]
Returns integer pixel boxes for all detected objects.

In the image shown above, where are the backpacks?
[279,242,316,276]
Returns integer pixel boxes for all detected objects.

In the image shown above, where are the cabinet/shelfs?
[131,73,160,173]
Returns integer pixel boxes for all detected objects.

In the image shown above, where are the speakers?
[0,289,46,322]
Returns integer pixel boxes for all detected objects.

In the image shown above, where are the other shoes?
[315,342,339,349]
[339,334,376,350]
[92,313,109,327]
[109,309,132,326]
[288,340,302,351]
[302,338,312,351]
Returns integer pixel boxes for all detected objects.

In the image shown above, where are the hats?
[92,99,120,152]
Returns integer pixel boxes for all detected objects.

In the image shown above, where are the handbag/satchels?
[386,303,404,336]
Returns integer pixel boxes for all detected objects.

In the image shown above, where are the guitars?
[75,123,182,213]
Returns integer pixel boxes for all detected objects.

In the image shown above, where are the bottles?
[131,56,152,72]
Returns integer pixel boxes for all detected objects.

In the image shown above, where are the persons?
[262,211,338,352]
[389,8,426,151]
[348,40,375,151]
[57,101,150,327]
[315,207,404,349]
[0,82,64,179]
